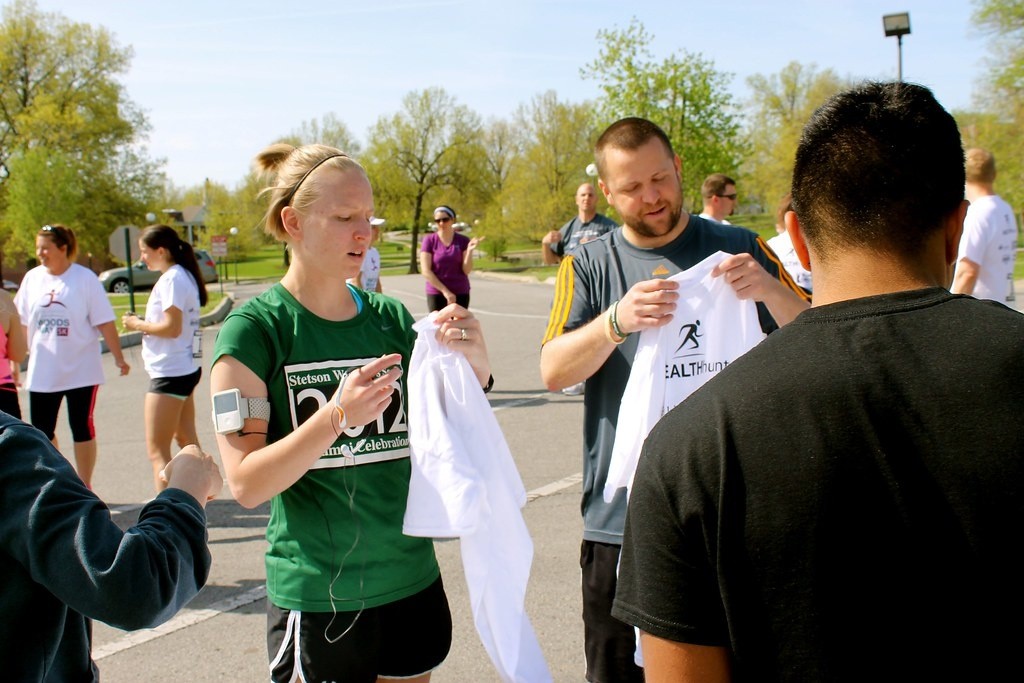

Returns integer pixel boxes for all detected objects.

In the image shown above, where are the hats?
[368,216,386,225]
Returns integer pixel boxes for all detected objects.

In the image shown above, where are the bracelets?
[611,301,632,338]
[483,375,494,394]
[606,305,627,344]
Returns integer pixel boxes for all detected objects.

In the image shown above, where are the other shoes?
[562,381,585,395]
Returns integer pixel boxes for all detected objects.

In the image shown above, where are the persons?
[419,205,486,313]
[539,116,811,683]
[0,408,222,683]
[121,225,209,495]
[0,286,29,420]
[207,136,496,683]
[345,215,386,295]
[13,224,130,492]
[611,80,1024,683]
[542,184,620,396]
[948,147,1018,307]
[694,173,737,225]
[759,191,812,294]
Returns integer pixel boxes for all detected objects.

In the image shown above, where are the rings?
[461,327,467,341]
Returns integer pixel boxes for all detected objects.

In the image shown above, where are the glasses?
[434,217,450,223]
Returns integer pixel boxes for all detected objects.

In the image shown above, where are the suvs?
[99,250,218,294]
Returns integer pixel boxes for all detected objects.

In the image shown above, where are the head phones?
[334,364,375,459]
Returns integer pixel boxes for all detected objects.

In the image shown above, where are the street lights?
[230,227,238,286]
